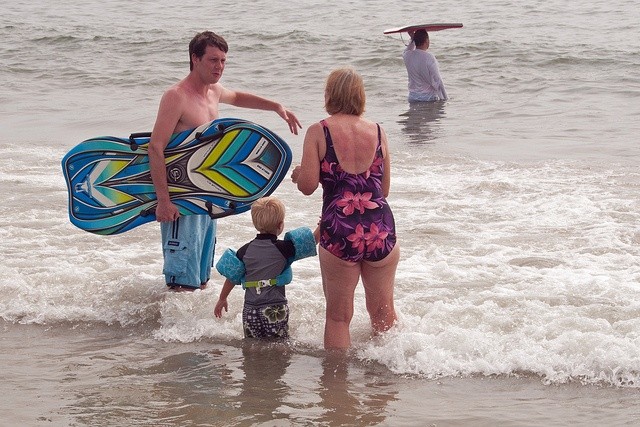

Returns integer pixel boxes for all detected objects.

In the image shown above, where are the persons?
[148,31,303,293]
[403,28,449,102]
[214,198,323,343]
[291,68,400,349]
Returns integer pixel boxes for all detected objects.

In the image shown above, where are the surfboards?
[61,116,294,236]
[382,20,464,36]
